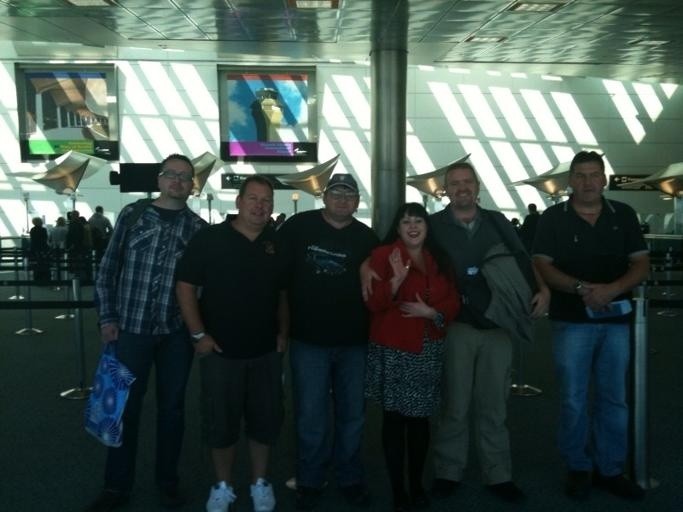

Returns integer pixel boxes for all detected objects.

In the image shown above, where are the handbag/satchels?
[82,340,136,449]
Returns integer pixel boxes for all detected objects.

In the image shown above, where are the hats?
[326,173,359,193]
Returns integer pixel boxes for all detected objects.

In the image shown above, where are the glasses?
[327,188,357,200]
[158,170,192,183]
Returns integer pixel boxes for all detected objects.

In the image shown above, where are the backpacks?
[83,220,100,249]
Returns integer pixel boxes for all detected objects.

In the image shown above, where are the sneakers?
[250,478,277,512]
[206,481,239,512]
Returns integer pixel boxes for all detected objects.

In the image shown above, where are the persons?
[364,203,460,511]
[78,216,97,286]
[89,206,114,284]
[65,212,84,280]
[48,218,68,282]
[30,218,51,280]
[281,173,381,503]
[94,153,210,511]
[176,173,289,509]
[524,204,540,247]
[532,151,651,504]
[360,164,551,511]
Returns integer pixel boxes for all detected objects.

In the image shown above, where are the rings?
[407,266,411,269]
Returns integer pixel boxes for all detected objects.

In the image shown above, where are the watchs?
[575,280,582,295]
[435,310,444,326]
[191,332,206,343]
